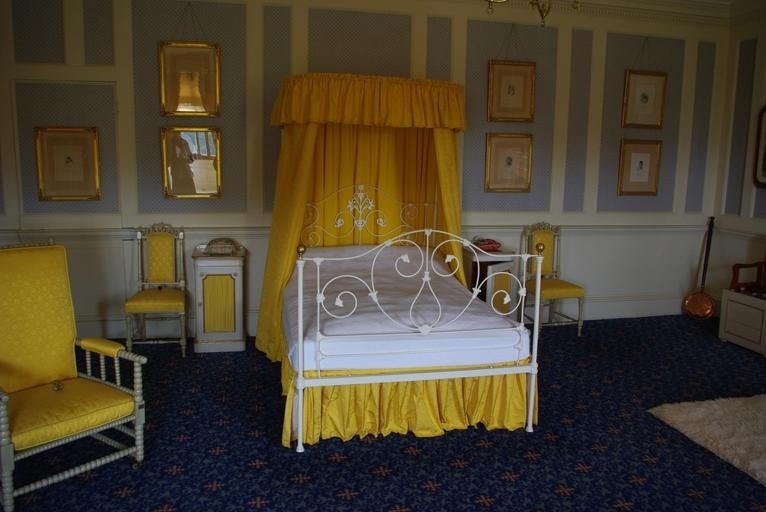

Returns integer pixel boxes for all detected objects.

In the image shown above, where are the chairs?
[122,221,191,358]
[1,241,149,511]
[516,220,584,339]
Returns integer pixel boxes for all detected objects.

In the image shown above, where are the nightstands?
[191,243,247,353]
[463,250,516,322]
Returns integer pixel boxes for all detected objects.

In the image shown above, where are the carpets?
[645,394,766,489]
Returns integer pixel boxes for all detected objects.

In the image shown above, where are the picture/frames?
[31,126,104,202]
[155,40,223,200]
[484,58,535,194]
[615,67,667,197]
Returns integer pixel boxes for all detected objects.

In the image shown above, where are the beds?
[269,182,544,455]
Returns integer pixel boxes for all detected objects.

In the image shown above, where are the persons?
[165,130,198,195]
